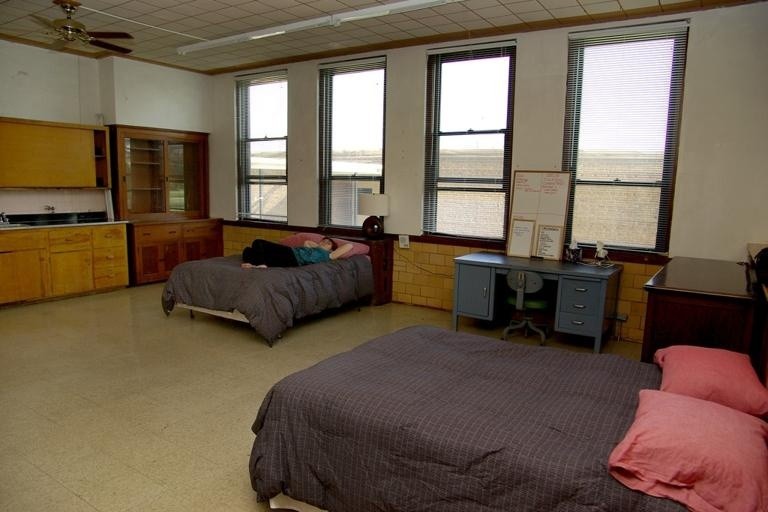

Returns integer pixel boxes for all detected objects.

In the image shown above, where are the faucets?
[0,211,6,222]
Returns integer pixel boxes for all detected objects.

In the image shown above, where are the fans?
[26,1,134,59]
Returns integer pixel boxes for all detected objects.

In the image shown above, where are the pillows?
[278,232,371,260]
[606,387,768,512]
[651,344,768,418]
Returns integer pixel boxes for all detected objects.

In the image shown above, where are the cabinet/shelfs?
[458,263,495,322]
[0,115,113,193]
[182,217,225,262]
[105,123,210,223]
[131,217,182,287]
[0,220,132,308]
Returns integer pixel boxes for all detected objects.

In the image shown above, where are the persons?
[240,236,354,270]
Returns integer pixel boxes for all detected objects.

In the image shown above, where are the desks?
[639,255,762,365]
[451,250,625,355]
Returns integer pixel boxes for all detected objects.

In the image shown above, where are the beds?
[246,323,765,512]
[161,255,376,348]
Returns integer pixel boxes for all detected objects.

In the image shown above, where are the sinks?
[0,223,30,229]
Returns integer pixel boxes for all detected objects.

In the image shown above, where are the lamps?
[175,15,334,57]
[356,191,389,242]
[334,0,465,28]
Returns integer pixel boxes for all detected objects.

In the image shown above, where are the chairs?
[499,269,547,348]
